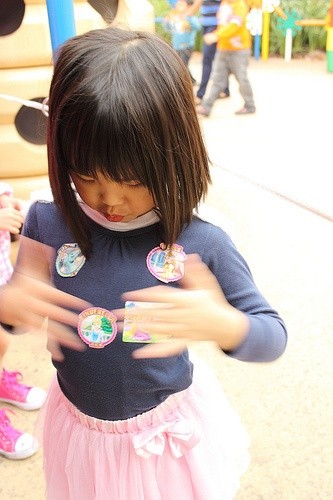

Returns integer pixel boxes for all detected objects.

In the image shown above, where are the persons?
[163,0,255,117]
[0,28,288,500]
[0,180,46,461]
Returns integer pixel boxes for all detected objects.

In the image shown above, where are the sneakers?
[0,368,48,411]
[0,407,40,460]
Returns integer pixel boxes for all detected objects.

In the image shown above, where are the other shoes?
[235,107,256,113]
[196,105,210,117]
